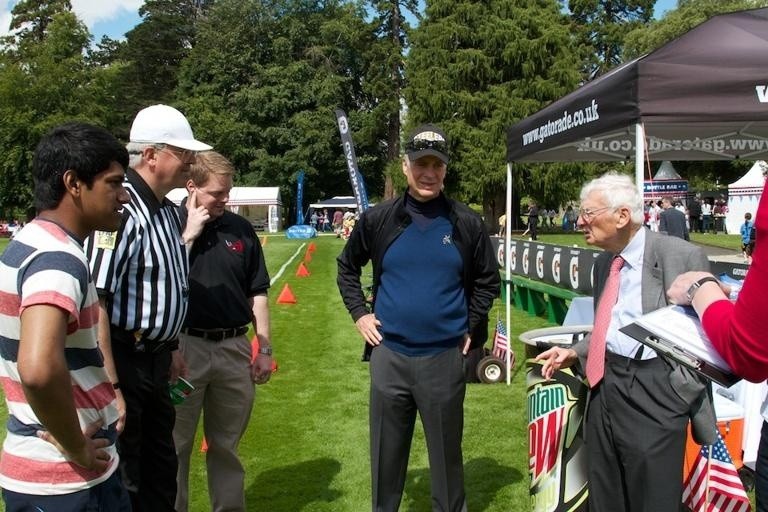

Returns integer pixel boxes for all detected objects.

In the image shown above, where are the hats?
[404,126,450,163]
[128,102,214,154]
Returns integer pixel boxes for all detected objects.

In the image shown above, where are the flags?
[492,313,515,370]
[681,420,752,512]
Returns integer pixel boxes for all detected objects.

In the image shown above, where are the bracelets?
[113,382,121,389]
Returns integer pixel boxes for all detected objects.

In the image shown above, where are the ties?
[584,258,624,387]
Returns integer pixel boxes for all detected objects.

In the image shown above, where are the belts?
[181,327,250,344]
[111,336,180,358]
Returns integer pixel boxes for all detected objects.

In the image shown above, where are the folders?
[618,280,745,389]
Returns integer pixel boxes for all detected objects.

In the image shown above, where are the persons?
[535,170,717,512]
[675,200,686,215]
[333,208,344,230]
[717,195,729,233]
[323,209,332,231]
[335,124,501,512]
[0,120,132,511]
[563,206,576,224]
[498,213,506,237]
[668,174,768,512]
[740,213,755,264]
[523,204,537,241]
[311,211,318,228]
[84,104,213,512]
[660,196,691,242]
[317,212,325,232]
[540,204,549,231]
[170,151,270,512]
[549,207,557,228]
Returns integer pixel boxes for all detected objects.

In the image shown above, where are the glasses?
[661,201,668,205]
[578,207,610,222]
[138,143,196,165]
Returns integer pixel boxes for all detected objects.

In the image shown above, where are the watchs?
[686,277,720,305]
[258,347,273,355]
